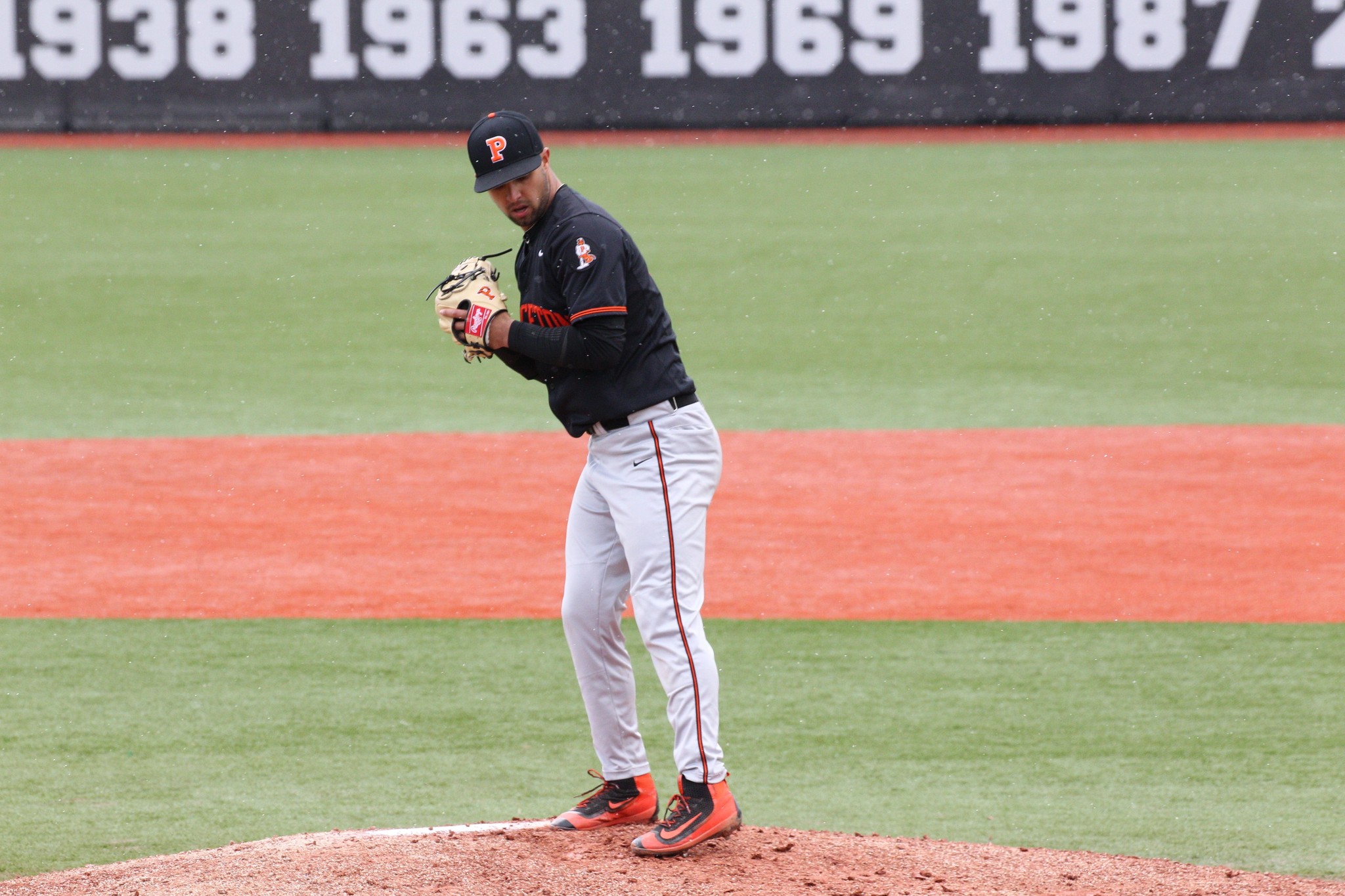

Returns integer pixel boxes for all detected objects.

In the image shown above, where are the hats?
[467,111,542,193]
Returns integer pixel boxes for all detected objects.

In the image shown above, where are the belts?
[587,392,698,436]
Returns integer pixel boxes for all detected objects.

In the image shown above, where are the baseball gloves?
[434,257,509,365]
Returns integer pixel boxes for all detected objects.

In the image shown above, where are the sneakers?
[631,771,742,861]
[551,769,658,830]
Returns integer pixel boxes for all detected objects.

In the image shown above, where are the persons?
[440,111,745,855]
